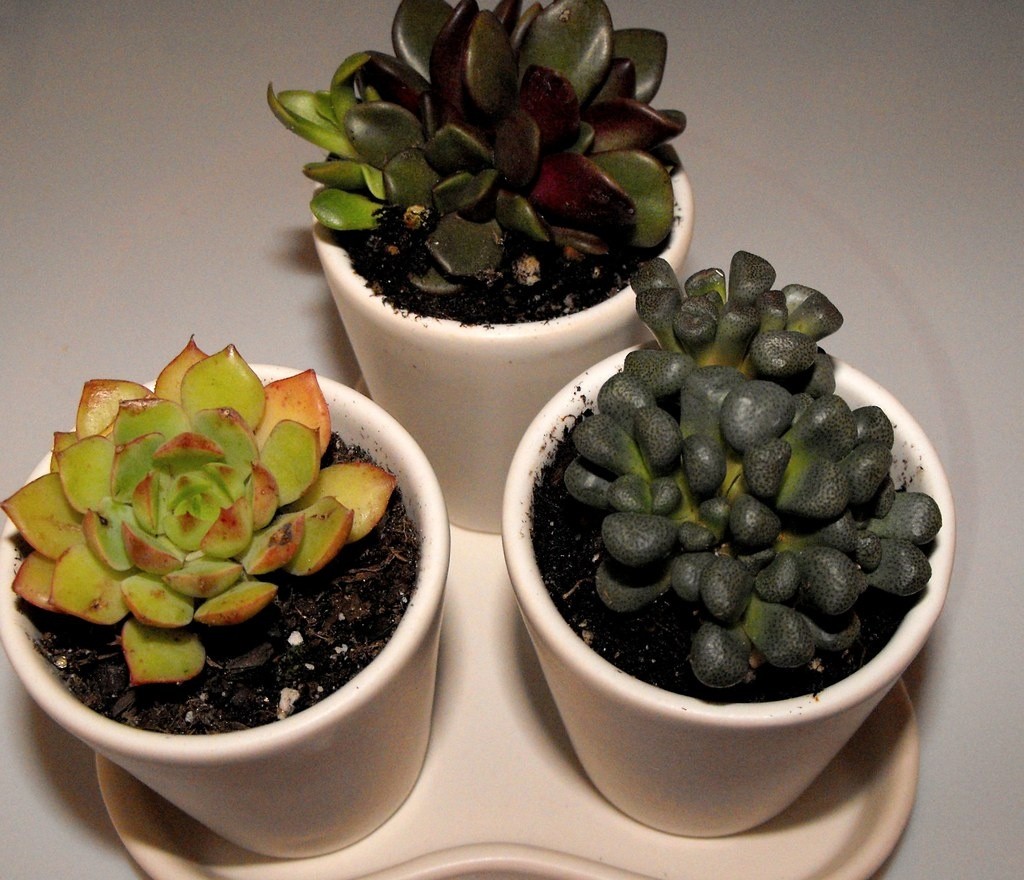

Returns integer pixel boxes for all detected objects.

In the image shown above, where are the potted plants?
[1,336,452,859]
[266,0,696,534]
[504,249,955,841]
